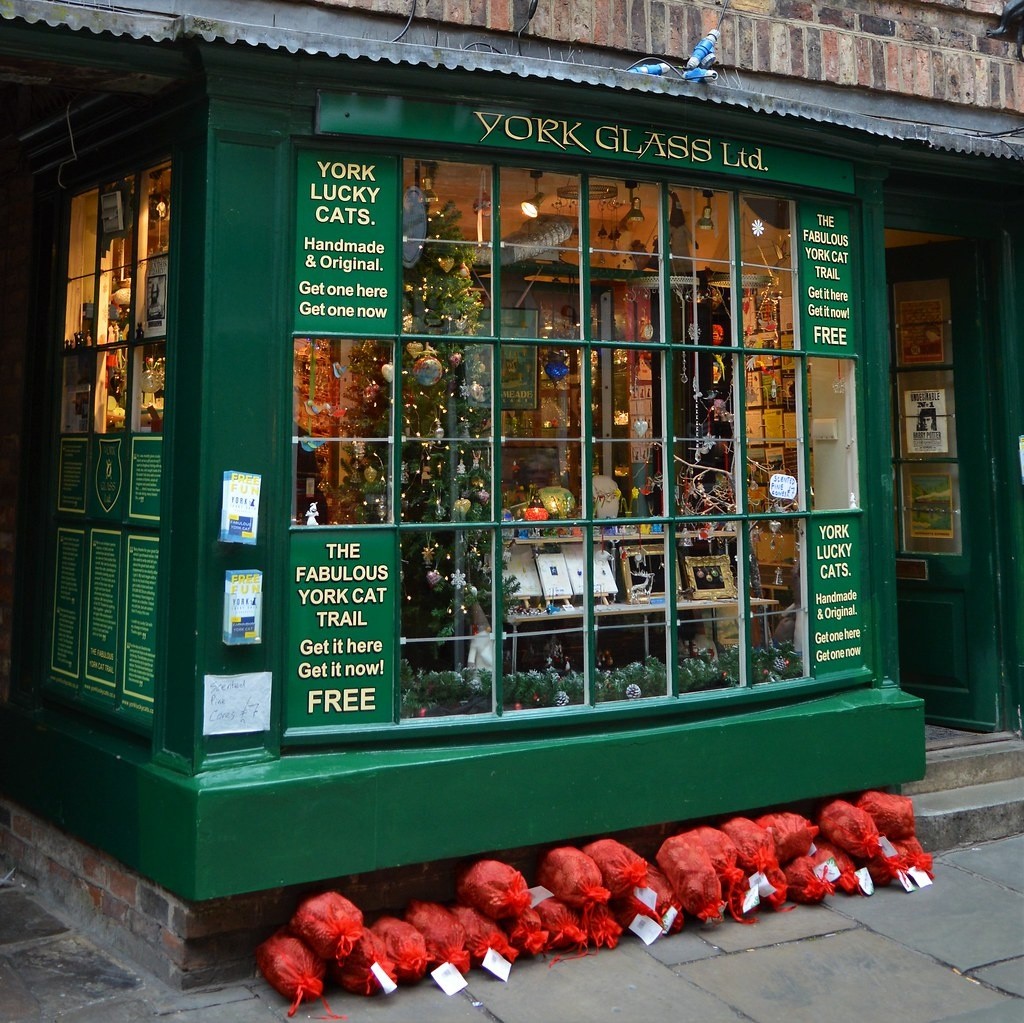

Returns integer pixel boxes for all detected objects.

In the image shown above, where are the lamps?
[417,177,439,203]
[520,192,547,219]
[627,198,645,223]
[695,206,714,230]
[524,493,548,521]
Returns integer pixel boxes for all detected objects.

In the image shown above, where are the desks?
[514,531,738,558]
[505,596,779,673]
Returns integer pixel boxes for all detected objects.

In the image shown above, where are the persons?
[920,409,936,431]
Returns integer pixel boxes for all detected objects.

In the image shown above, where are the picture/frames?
[684,554,738,600]
[618,545,684,606]
[463,306,540,411]
